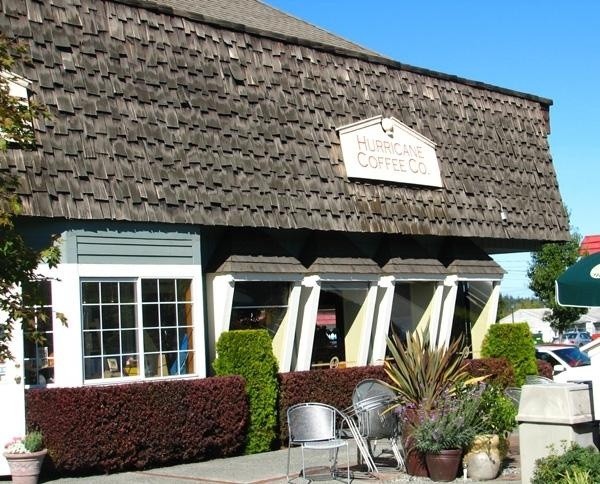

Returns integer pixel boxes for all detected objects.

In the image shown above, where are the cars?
[552,331,592,346]
[535,345,592,384]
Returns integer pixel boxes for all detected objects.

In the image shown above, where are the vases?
[425,447,463,483]
[3,446,48,484]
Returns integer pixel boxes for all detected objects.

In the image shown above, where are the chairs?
[524,373,555,385]
[503,384,521,407]
[281,378,413,484]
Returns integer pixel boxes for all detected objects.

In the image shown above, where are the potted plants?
[377,321,492,477]
[463,383,518,482]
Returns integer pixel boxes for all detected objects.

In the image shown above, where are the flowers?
[393,379,489,468]
[4,429,46,455]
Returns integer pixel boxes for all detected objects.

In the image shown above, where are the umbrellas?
[555,251,600,308]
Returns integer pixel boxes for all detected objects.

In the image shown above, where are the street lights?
[510,303,515,324]
